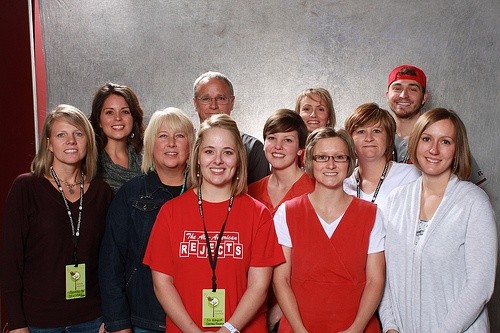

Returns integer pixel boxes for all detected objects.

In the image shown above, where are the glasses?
[195,94,233,106]
[311,153,351,162]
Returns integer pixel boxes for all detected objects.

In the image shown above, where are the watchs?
[223,322,240,333]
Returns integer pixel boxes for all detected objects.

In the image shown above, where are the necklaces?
[179,169,188,195]
[356,162,389,203]
[50,172,81,194]
[393,145,409,164]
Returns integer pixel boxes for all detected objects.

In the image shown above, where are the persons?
[272,126,386,333]
[98,107,196,333]
[0,104,115,333]
[378,108,498,333]
[89,82,153,194]
[192,71,270,184]
[142,113,287,333]
[386,65,487,186]
[247,109,315,333]
[296,87,336,167]
[343,102,422,207]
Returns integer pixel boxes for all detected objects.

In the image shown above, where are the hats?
[388,63,427,90]
